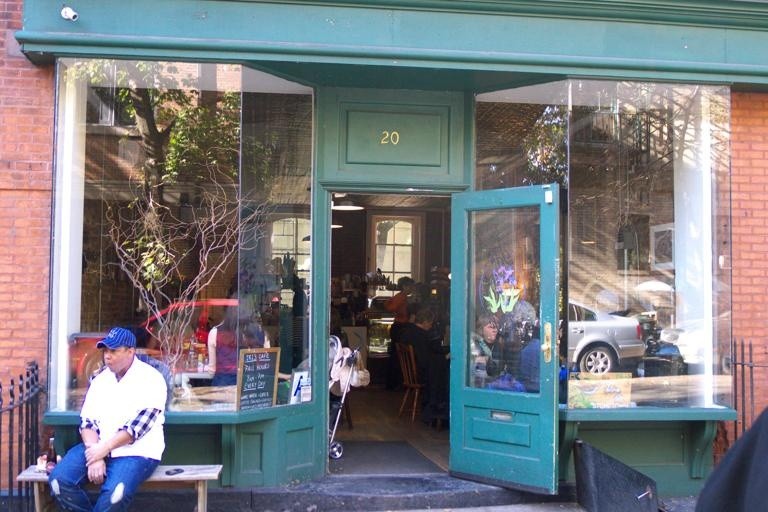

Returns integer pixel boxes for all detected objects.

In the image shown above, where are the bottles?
[185,337,196,373]
[46,438,57,475]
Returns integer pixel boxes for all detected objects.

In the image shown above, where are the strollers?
[298,335,370,460]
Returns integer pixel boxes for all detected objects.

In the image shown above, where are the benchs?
[15,462,224,512]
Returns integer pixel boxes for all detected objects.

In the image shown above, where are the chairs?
[393,342,426,422]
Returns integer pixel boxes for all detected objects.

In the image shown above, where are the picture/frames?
[649,221,676,272]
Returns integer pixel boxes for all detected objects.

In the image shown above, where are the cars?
[68,299,238,386]
[568,298,645,373]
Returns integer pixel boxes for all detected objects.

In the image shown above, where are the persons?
[48,327,167,512]
[207,290,270,386]
[695,408,768,512]
[290,277,541,427]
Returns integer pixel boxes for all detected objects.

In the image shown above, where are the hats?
[96,327,137,349]
